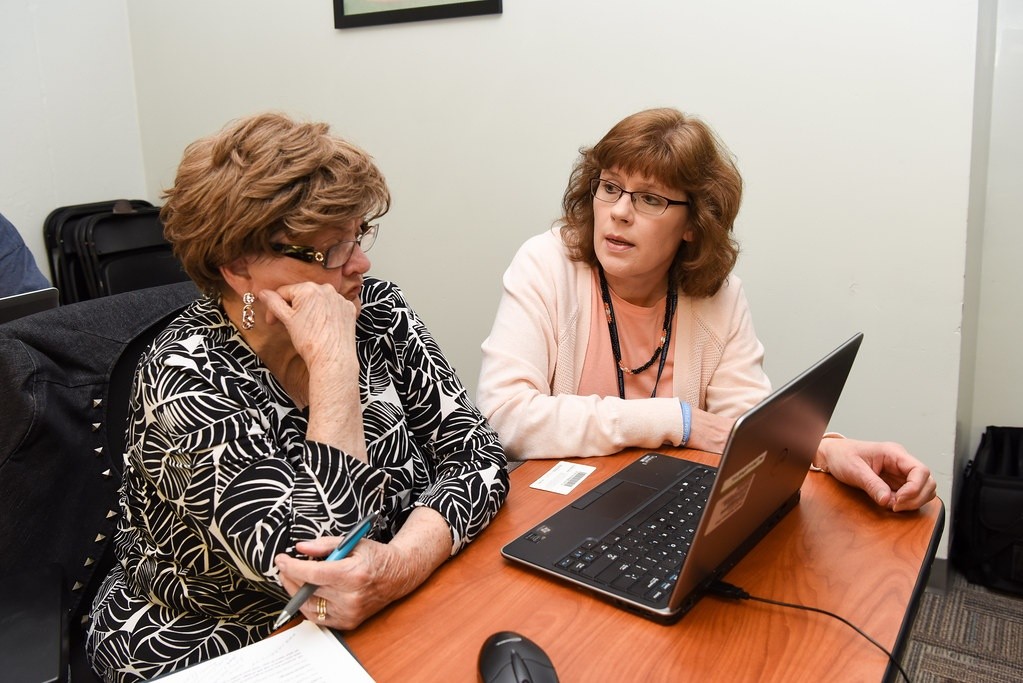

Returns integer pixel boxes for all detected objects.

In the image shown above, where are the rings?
[317,597,327,620]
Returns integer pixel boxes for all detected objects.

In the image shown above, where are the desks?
[254,445,944,683]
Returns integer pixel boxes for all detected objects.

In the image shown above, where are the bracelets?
[680,401,691,445]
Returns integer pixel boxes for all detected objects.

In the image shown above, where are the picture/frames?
[333,0,502,29]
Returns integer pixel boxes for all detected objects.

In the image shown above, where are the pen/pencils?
[272,510,381,630]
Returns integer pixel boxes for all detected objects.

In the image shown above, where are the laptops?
[501,332,864,626]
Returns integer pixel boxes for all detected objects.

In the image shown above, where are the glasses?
[243,220,378,269]
[589,178,692,215]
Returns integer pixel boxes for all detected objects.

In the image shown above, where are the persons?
[86,114,510,683]
[0,213,50,299]
[478,108,937,512]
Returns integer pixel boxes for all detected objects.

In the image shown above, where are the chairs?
[0,198,213,683]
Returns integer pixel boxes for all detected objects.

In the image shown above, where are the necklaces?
[597,265,673,373]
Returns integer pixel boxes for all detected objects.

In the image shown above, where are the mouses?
[477,631,560,683]
